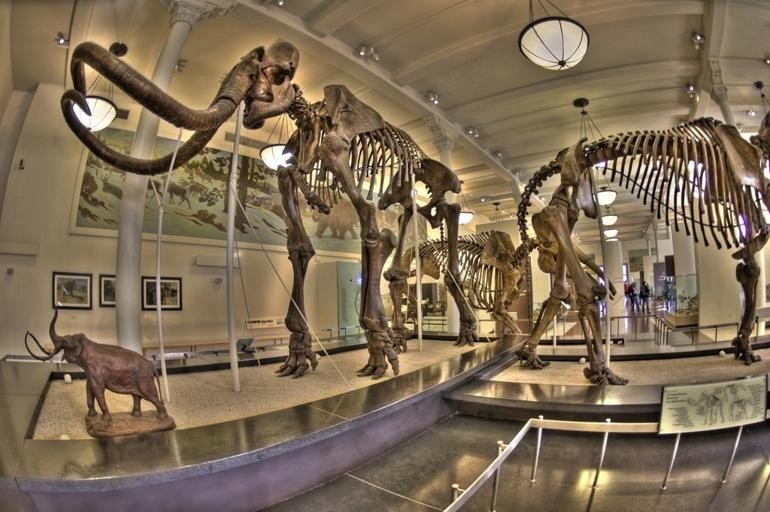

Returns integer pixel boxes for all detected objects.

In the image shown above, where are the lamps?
[592,166,619,239]
[455,185,474,226]
[518,0,590,71]
[69,42,129,132]
[261,111,295,172]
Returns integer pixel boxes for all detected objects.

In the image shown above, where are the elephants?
[24,305,171,423]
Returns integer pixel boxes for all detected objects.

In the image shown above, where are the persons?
[598,279,651,314]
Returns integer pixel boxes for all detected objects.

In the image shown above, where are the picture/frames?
[52,270,183,311]
